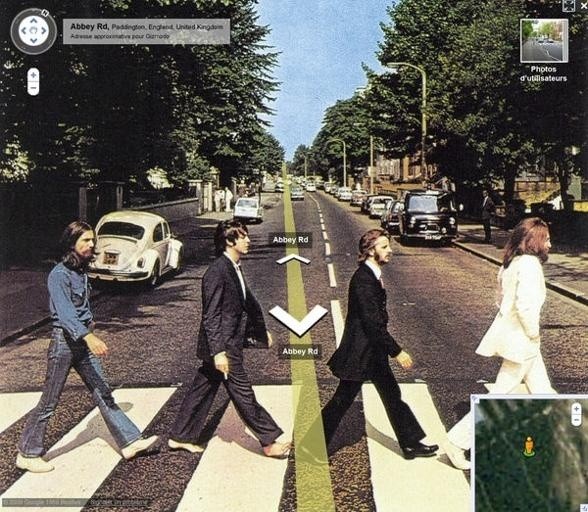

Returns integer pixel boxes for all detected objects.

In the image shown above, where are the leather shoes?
[442,438,471,470]
[296,446,331,465]
[403,443,439,459]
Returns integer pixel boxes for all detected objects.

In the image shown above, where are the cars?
[273,172,325,201]
[531,34,556,43]
[324,183,400,233]
[86,209,185,288]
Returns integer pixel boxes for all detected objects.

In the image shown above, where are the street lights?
[387,62,428,185]
[327,138,348,187]
[354,122,376,195]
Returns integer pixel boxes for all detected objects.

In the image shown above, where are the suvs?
[232,197,265,223]
[398,187,459,247]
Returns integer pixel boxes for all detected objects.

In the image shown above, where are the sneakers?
[15,453,54,472]
[121,435,159,459]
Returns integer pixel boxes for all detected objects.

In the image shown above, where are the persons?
[480,189,495,244]
[225,186,233,210]
[14,220,160,474]
[301,228,439,466]
[170,218,294,457]
[442,219,560,471]
